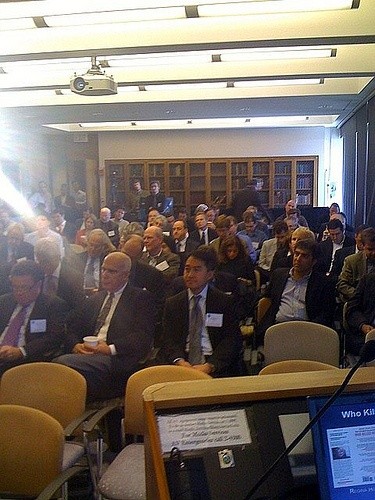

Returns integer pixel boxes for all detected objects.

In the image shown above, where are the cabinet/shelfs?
[104,155,319,216]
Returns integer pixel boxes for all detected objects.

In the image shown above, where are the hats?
[287,208,301,215]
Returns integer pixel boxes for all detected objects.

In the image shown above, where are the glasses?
[12,278,38,294]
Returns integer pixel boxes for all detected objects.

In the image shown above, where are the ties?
[199,231,206,245]
[92,292,115,337]
[175,242,181,253]
[148,257,157,266]
[0,304,30,348]
[188,294,203,367]
[85,256,97,288]
[46,275,56,296]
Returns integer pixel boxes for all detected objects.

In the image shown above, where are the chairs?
[0,321,340,500]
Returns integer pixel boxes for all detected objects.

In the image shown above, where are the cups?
[83,336,100,347]
[278,413,316,477]
[207,222,212,228]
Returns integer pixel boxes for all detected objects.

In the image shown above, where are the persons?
[0,178,375,407]
[336,448,350,459]
[160,250,250,379]
[51,253,157,401]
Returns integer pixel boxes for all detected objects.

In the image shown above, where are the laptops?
[307,390,375,500]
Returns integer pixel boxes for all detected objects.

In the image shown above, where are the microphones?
[244,339,375,500]
